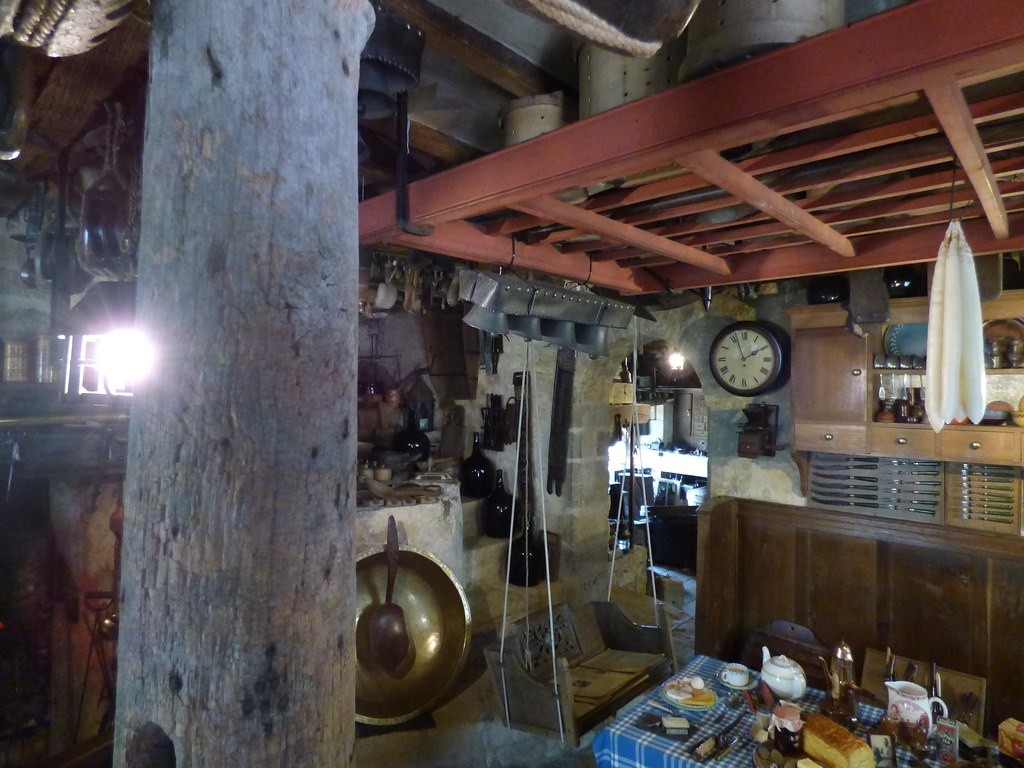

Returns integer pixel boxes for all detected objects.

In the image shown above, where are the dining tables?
[591,655,1024,768]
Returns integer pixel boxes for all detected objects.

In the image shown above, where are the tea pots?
[819,640,876,729]
[762,646,807,700]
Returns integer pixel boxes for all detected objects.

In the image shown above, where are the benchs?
[484,600,679,750]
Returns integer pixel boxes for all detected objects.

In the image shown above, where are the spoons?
[648,699,684,717]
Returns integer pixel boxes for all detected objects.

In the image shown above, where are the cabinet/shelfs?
[786,290,1024,535]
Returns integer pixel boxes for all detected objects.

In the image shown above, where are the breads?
[797,757,822,768]
[680,692,716,705]
[799,711,876,768]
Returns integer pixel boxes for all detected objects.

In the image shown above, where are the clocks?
[710,321,783,396]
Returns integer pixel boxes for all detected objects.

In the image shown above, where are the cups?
[721,663,749,686]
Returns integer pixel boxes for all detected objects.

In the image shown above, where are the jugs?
[884,680,949,738]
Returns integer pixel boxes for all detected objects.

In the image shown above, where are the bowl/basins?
[984,410,1008,419]
[1010,411,1024,427]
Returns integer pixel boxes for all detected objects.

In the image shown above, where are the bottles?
[460,432,494,498]
[396,404,430,462]
[483,470,518,538]
[508,514,544,586]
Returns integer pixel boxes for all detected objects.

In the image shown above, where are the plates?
[981,418,1008,426]
[753,740,783,768]
[981,318,1024,369]
[883,323,928,357]
[716,668,758,689]
[659,684,718,710]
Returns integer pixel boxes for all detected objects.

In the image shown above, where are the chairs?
[742,619,832,691]
[861,647,986,737]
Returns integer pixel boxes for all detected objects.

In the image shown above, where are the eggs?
[691,677,704,689]
